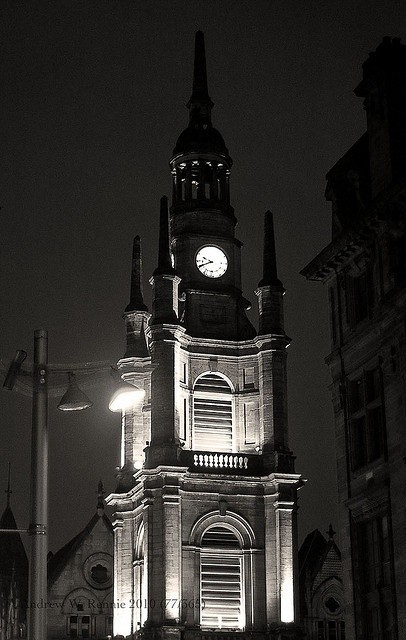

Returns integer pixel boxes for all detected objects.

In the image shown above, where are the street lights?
[28,358,146,640]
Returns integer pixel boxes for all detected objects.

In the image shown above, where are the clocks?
[195,244,229,279]
